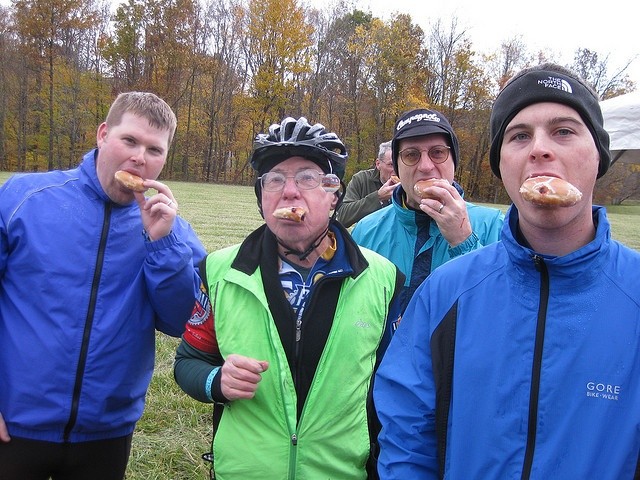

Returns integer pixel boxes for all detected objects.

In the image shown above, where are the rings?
[437,204,445,212]
[164,200,174,209]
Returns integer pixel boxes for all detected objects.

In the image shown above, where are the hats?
[392,109,459,178]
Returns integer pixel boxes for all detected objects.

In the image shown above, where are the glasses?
[257,169,324,192]
[378,157,394,168]
[398,145,451,166]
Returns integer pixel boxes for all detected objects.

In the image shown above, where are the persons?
[1,90,205,480]
[349,110,505,311]
[173,116,406,480]
[373,64,640,480]
[338,140,401,227]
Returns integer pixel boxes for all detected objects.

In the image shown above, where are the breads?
[115,170,148,193]
[272,206,308,223]
[413,178,447,199]
[520,176,582,208]
[391,175,401,183]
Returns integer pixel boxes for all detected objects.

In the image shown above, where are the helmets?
[250,117,349,205]
